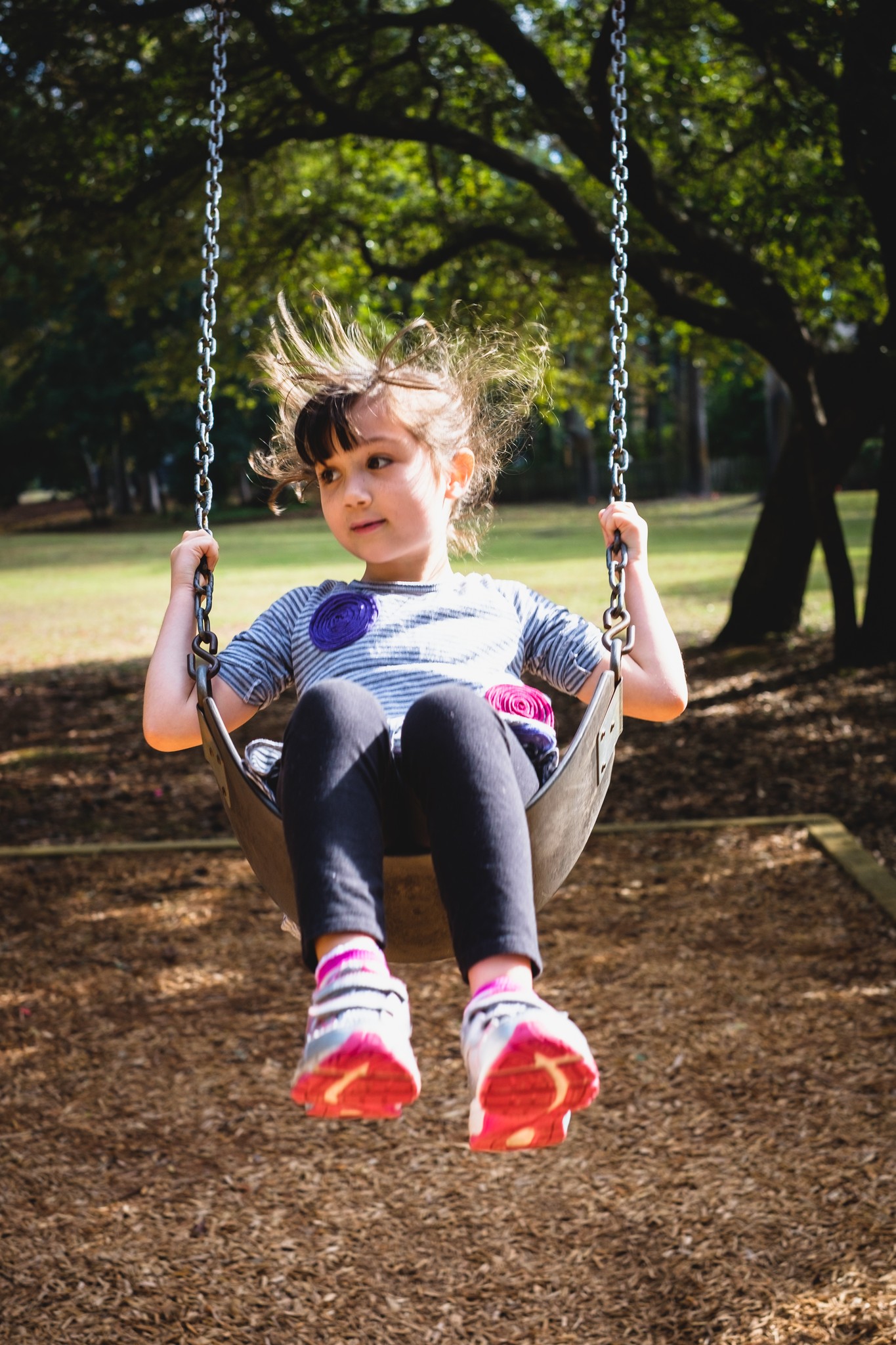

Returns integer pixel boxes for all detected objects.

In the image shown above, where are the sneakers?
[459,991,600,1151]
[288,971,420,1119]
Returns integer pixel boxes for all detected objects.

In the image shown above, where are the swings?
[189,1,637,964]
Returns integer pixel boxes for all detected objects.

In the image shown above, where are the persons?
[144,288,688,1153]
